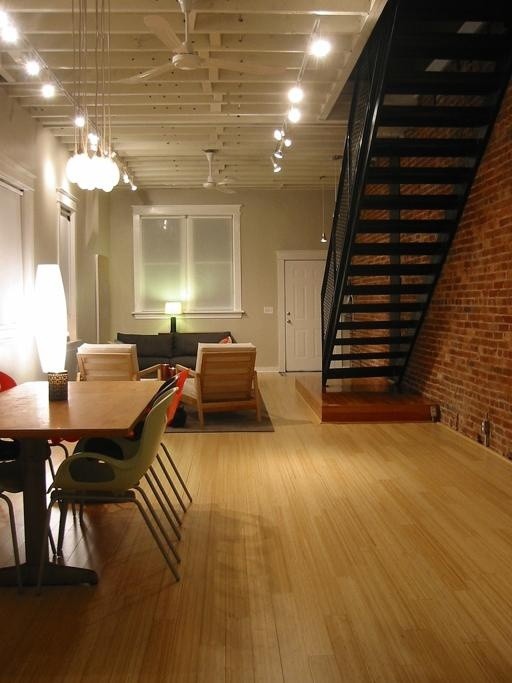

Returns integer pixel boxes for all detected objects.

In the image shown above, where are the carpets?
[165,388,274,432]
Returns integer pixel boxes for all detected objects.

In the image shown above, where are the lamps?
[269,19,331,173]
[0,5,139,191]
[165,301,182,333]
[320,177,327,243]
[66,0,119,192]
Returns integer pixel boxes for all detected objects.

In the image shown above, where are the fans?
[127,0,267,84]
[202,149,237,195]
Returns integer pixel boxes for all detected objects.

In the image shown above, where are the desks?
[0,378,169,594]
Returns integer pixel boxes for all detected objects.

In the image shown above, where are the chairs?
[0,370,71,590]
[33,370,191,594]
[175,342,264,429]
[76,343,162,381]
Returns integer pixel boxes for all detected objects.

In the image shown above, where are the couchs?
[117,332,237,378]
